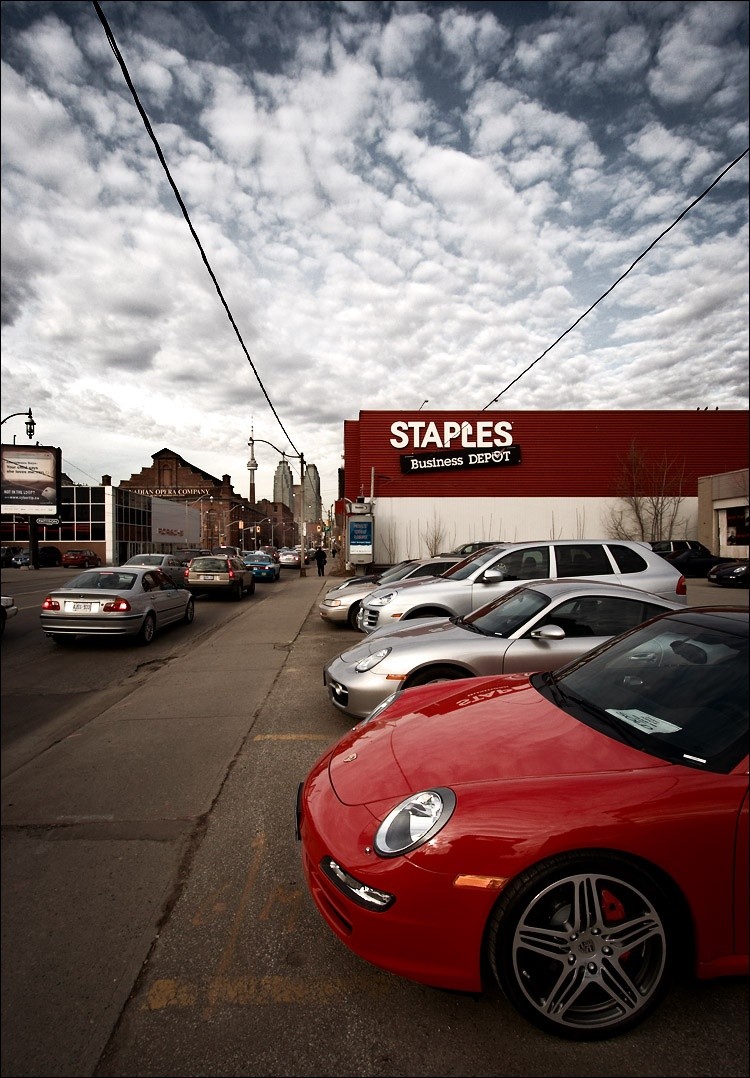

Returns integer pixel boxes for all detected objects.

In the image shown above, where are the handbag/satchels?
[322,559,327,565]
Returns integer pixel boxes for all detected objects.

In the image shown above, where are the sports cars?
[707,562,750,588]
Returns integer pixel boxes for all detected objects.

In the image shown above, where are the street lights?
[247,437,306,577]
[272,522,285,548]
[255,518,271,550]
[283,528,295,544]
[222,504,244,546]
[185,494,213,548]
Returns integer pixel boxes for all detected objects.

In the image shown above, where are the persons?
[332,546,337,558]
[728,531,737,545]
[314,546,327,577]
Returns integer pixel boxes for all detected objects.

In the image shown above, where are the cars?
[11,546,62,567]
[652,541,710,558]
[183,556,255,600]
[40,554,195,645]
[174,545,318,568]
[242,551,281,582]
[1,596,18,633]
[0,546,20,568]
[296,605,750,1042]
[318,540,688,635]
[61,550,101,568]
[663,551,736,578]
[323,579,687,719]
